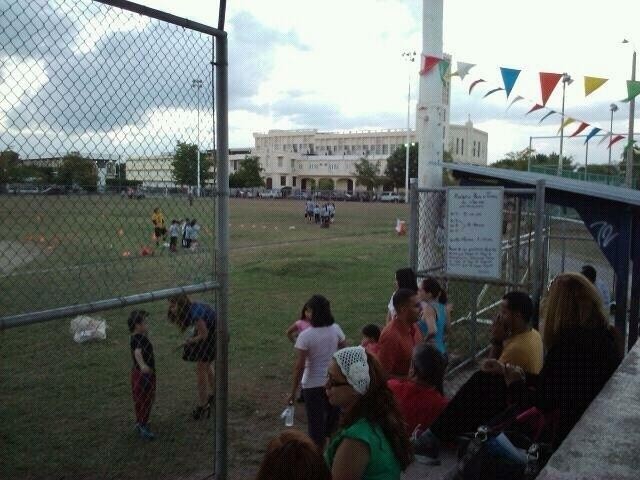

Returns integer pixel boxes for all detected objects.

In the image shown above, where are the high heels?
[194,393,215,419]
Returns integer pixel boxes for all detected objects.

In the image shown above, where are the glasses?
[326,371,350,390]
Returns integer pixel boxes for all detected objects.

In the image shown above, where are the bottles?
[285,401,295,427]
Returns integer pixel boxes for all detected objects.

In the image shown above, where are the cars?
[41,184,65,196]
[377,192,402,203]
[181,186,377,202]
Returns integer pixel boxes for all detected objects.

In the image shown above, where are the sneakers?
[407,423,440,466]
[136,421,154,441]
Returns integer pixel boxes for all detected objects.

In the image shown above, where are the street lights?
[620,37,640,190]
[607,103,620,185]
[557,72,575,176]
[399,49,422,203]
[116,152,125,195]
[190,75,208,198]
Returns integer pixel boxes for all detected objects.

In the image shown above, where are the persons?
[128,310,156,439]
[255,264,620,480]
[152,208,201,252]
[167,294,229,420]
[305,197,336,224]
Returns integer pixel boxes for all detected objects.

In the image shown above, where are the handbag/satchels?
[446,409,544,480]
